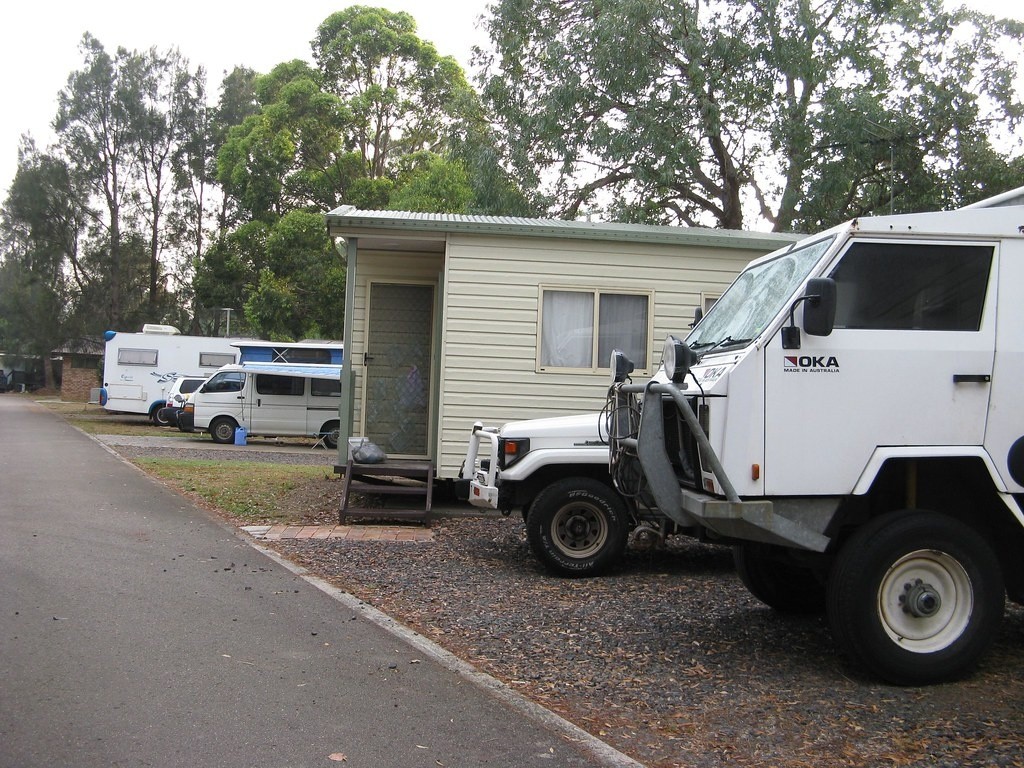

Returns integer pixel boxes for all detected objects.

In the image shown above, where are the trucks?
[99,325,274,428]
[600,205,1024,689]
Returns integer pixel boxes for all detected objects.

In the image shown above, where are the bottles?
[234,427,248,446]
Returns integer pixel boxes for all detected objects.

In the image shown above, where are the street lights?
[222,308,234,337]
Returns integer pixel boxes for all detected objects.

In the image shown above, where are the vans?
[176,361,340,450]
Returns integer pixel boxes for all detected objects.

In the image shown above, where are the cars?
[160,375,244,434]
[455,413,662,583]
